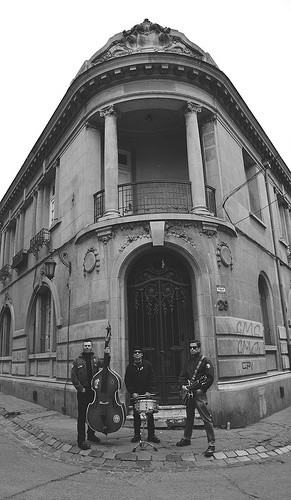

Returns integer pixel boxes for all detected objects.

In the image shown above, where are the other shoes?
[148,435,160,443]
[78,441,90,449]
[206,445,215,455]
[176,439,191,447]
[87,434,100,442]
[132,434,141,443]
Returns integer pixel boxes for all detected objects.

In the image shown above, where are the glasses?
[134,352,142,355]
[189,346,199,350]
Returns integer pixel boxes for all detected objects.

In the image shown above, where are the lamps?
[50,248,72,276]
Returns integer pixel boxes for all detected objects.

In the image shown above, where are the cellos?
[86,324,126,441]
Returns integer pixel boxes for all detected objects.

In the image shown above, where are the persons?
[71,340,112,450]
[124,347,160,444]
[176,340,216,456]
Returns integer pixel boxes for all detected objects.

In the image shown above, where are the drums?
[134,399,159,413]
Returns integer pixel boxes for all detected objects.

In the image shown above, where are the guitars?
[181,374,208,404]
